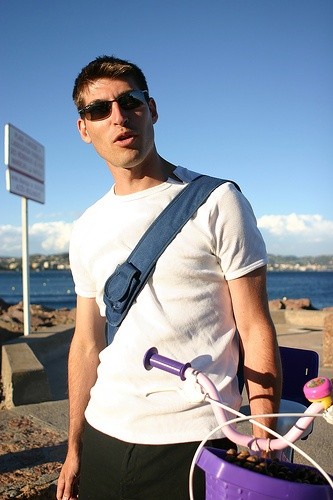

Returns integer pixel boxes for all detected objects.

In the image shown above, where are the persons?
[55,57,284,500]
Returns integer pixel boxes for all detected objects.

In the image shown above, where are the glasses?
[77,90,148,122]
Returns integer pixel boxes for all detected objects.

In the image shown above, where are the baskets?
[196,447,333,500]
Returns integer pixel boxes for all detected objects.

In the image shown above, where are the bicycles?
[141,346,333,500]
